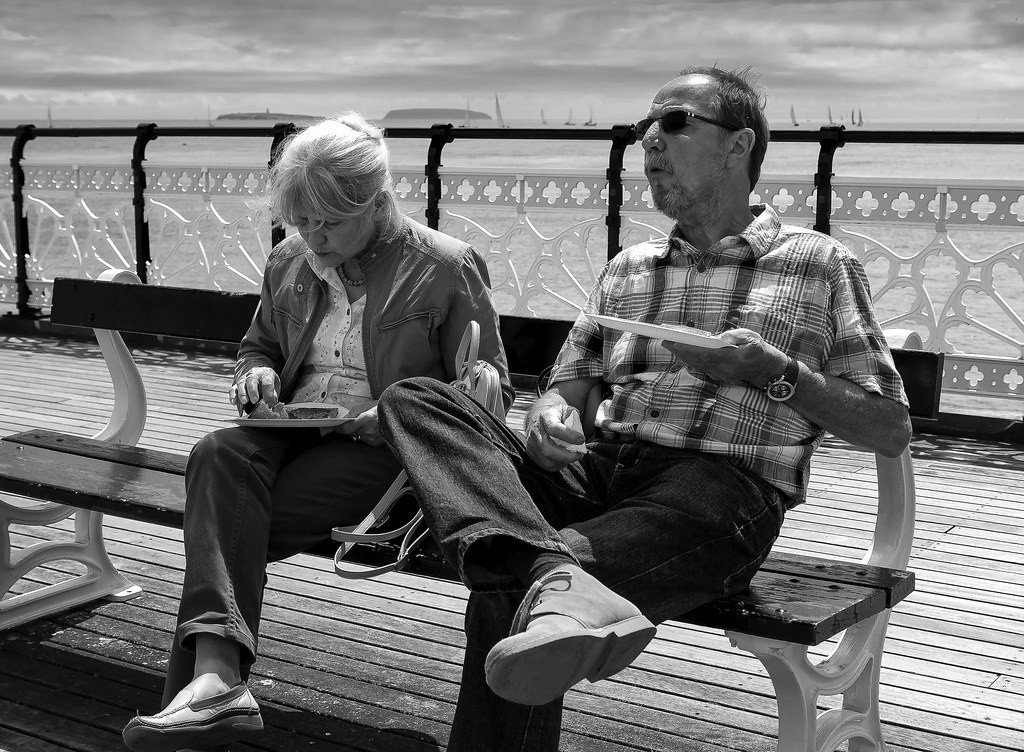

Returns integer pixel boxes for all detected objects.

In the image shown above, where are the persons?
[378,67,914,752]
[120,112,514,752]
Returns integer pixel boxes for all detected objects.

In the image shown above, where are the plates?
[583,313,741,349]
[221,403,357,427]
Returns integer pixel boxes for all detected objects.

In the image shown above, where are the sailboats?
[458,100,472,128]
[850,109,857,125]
[790,104,799,126]
[584,105,598,127]
[563,109,576,125]
[857,109,864,127]
[827,105,836,127]
[494,92,510,128]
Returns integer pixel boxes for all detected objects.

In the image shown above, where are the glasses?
[630,111,735,136]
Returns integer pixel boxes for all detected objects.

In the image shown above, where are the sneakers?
[484,564,658,705]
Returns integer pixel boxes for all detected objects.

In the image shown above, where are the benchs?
[0,278,945,752]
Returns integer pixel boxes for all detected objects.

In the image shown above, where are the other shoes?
[123,680,263,752]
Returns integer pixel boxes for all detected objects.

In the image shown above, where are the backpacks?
[330,320,507,580]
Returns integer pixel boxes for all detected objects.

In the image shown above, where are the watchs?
[764,356,800,403]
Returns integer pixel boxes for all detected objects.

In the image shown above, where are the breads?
[247,399,289,419]
[287,407,338,419]
